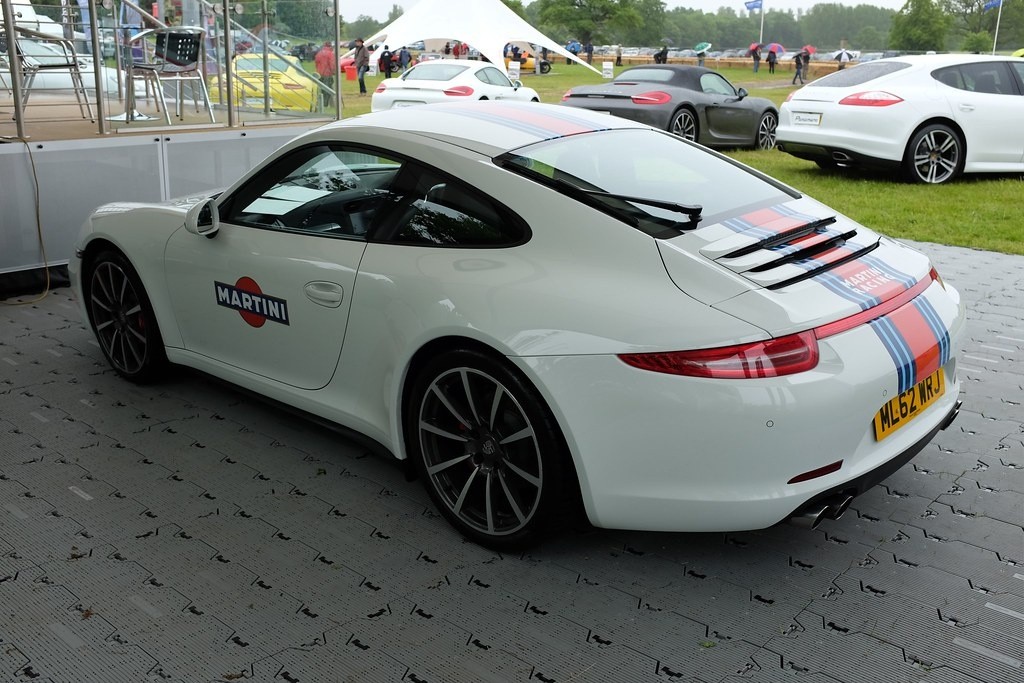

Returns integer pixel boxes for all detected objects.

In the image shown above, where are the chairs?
[940,72,959,88]
[125,25,215,126]
[12,28,96,124]
[974,73,1000,94]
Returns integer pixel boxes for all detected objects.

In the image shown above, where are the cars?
[563,64,780,148]
[774,53,1024,185]
[367,59,544,107]
[59,97,974,560]
[209,48,322,116]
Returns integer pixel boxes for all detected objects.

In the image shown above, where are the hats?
[568,41,571,43]
[355,39,364,43]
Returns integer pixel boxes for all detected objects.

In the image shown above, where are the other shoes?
[793,83,796,84]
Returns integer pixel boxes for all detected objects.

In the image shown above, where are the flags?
[744,0,762,9]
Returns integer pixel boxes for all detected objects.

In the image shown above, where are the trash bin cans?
[345,66,358,79]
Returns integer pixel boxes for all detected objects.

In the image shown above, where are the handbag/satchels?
[365,66,369,72]
[796,64,802,69]
[775,60,778,64]
[766,55,769,62]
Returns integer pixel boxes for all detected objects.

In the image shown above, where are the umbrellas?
[268,39,290,48]
[800,45,815,55]
[744,40,764,57]
[763,43,786,52]
[661,38,674,44]
[791,50,805,58]
[833,48,854,62]
[693,42,712,54]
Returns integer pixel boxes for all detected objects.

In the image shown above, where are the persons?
[504,42,548,60]
[300,38,412,107]
[765,51,777,74]
[803,49,810,79]
[792,56,804,84]
[444,41,470,60]
[697,52,706,67]
[565,38,668,66]
[751,46,762,73]
[838,61,845,70]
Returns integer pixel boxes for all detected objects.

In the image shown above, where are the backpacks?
[697,52,703,57]
